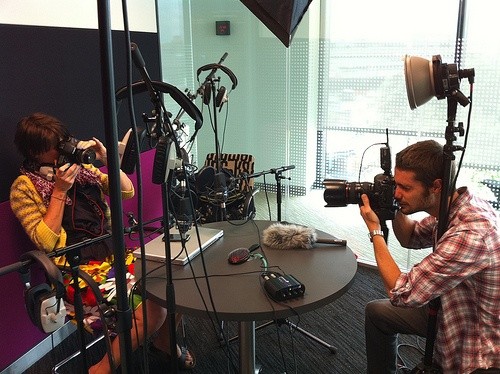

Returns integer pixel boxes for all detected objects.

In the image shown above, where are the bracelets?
[51,195,65,201]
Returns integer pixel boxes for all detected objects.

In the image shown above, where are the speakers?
[167,122,189,169]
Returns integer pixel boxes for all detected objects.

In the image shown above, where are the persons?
[10,112,196,374]
[360,140,500,374]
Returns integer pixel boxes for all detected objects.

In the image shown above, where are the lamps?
[403,53,475,111]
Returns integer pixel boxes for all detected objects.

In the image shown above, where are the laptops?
[132,226,223,267]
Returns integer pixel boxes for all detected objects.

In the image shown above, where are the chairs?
[191,151,256,220]
[45,227,187,374]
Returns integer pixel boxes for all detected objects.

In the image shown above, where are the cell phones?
[162,234,191,242]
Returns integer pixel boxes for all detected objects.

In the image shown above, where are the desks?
[135,219,358,374]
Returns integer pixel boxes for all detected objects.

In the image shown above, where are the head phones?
[196,63,237,108]
[20,250,65,332]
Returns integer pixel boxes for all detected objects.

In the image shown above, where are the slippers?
[147,342,195,370]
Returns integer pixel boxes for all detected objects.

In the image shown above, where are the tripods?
[219,165,338,353]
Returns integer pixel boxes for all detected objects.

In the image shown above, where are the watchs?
[368,230,384,243]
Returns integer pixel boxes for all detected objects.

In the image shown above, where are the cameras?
[55,135,96,167]
[322,146,401,220]
